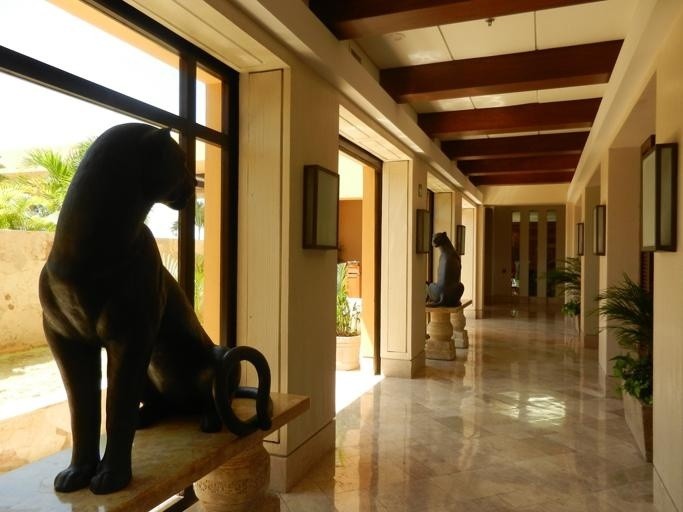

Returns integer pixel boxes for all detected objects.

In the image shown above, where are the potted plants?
[534,257,581,334]
[585,271,653,462]
[336,262,361,370]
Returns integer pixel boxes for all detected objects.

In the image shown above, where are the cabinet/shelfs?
[345,262,361,298]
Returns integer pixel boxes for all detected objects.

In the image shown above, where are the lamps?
[592,205,605,255]
[640,143,677,252]
[302,165,339,249]
[576,223,584,255]
[416,209,431,253]
[457,225,465,255]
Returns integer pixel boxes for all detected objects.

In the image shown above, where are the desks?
[425,299,472,360]
[0,391,311,512]
